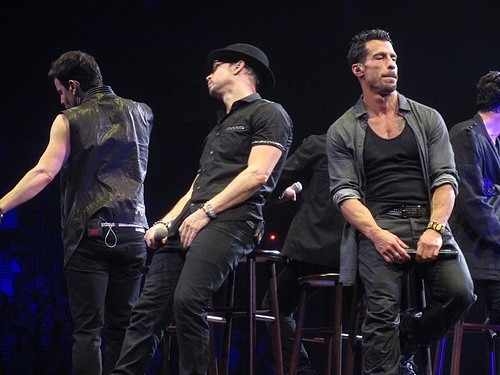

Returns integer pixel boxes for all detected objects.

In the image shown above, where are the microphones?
[278,181,302,200]
[154,227,167,242]
[404,249,458,257]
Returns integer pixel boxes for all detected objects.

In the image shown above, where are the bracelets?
[200,201,217,221]
[427,220,446,236]
[0,208,4,219]
[153,221,169,231]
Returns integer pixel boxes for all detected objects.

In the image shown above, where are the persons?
[326,30,477,375]
[0,51,154,375]
[449,72,500,333]
[113,43,294,375]
[261,133,348,375]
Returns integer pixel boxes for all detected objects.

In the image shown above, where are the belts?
[384,204,427,219]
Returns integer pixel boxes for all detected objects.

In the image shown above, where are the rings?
[393,253,400,259]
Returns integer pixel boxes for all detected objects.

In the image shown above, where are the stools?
[433,274,500,375]
[350,261,433,375]
[206,250,285,375]
[288,278,360,375]
[140,266,219,375]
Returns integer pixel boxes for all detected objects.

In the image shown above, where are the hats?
[208,44,276,96]
